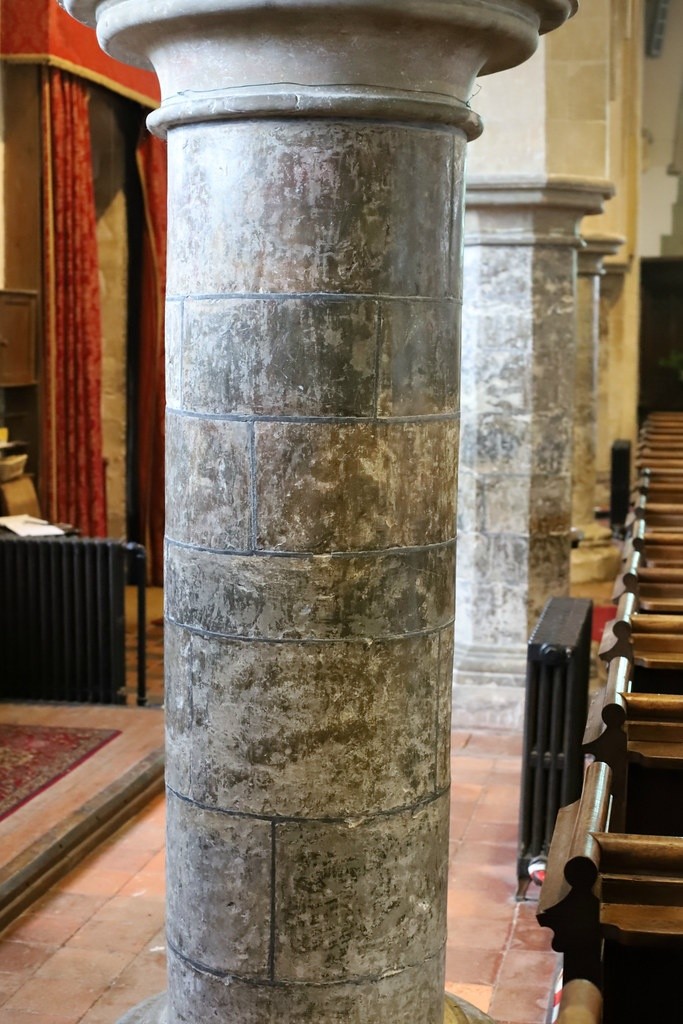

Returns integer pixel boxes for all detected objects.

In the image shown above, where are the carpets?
[0,725,122,820]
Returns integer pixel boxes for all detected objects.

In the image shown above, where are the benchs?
[580,412,682,943]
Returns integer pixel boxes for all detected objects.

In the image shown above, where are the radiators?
[515,597,595,901]
[607,438,632,537]
[0,535,148,704]
